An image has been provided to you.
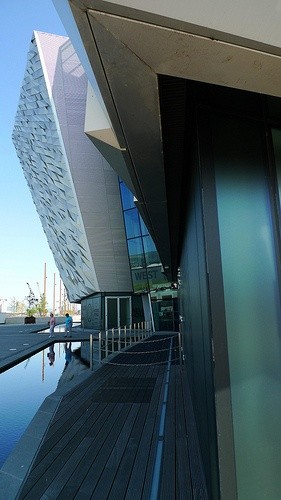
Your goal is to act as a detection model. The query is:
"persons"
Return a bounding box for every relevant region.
[47,343,55,366]
[48,313,56,338]
[63,313,73,338]
[65,341,72,366]
